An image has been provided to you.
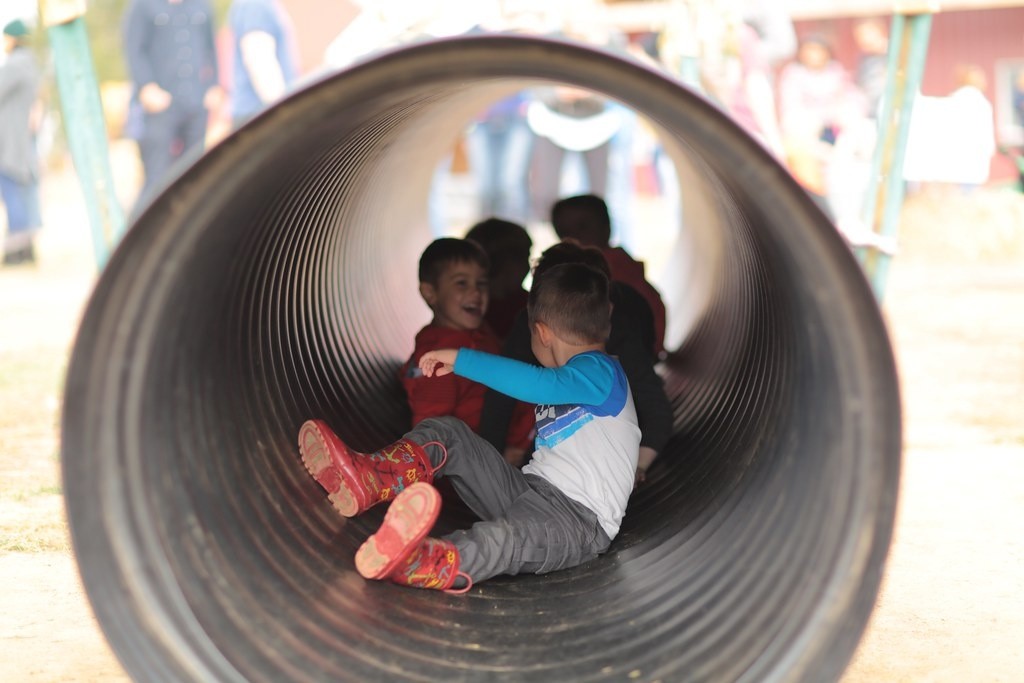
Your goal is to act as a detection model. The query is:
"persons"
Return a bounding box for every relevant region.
[648,0,1024,263]
[123,0,222,215]
[0,18,44,265]
[400,195,675,502]
[297,263,642,597]
[228,1,292,134]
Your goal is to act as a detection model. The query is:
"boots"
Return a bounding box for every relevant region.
[298,419,447,518]
[355,480,472,593]
